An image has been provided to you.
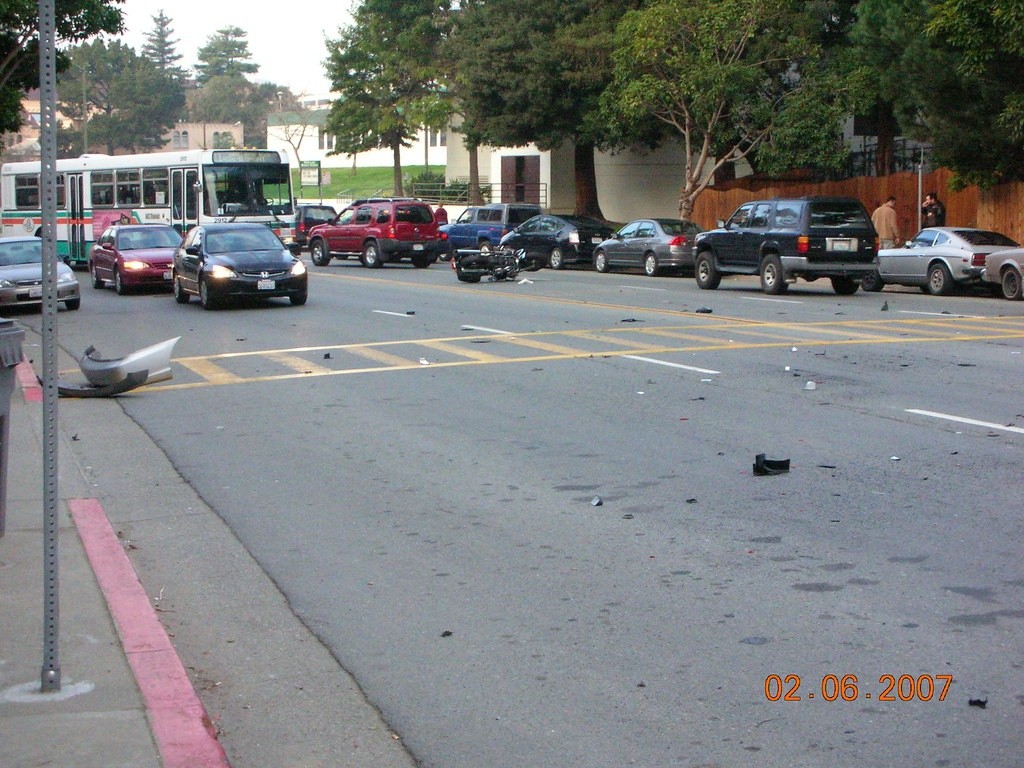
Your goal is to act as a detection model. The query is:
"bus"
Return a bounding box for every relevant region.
[1,148,299,264]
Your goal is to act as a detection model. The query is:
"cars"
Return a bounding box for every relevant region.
[591,218,707,277]
[88,223,184,296]
[499,213,616,271]
[862,226,1020,296]
[171,223,308,311]
[984,246,1023,301]
[0,235,81,317]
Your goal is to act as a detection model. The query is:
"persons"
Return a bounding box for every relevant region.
[434,203,448,225]
[871,196,900,249]
[921,192,945,230]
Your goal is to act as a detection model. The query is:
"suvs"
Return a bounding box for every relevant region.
[294,202,337,247]
[436,202,542,262]
[691,196,881,295]
[306,196,442,271]
